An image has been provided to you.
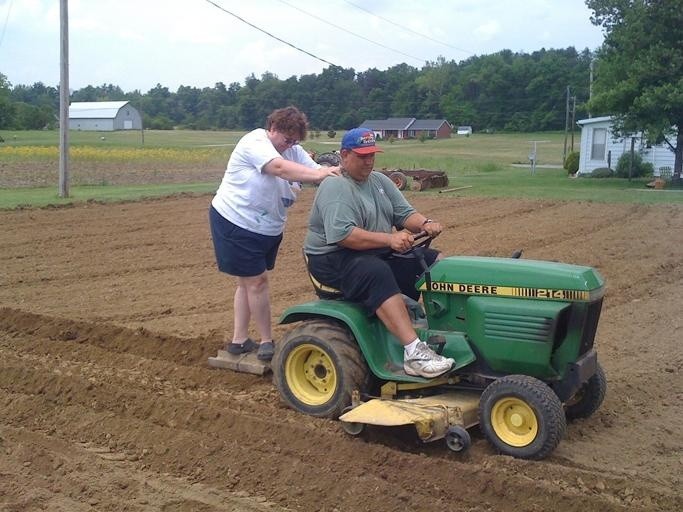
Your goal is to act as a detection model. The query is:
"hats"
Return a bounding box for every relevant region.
[341,127,384,155]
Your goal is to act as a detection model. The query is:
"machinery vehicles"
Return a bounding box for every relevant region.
[313,152,449,192]
[269,229,607,460]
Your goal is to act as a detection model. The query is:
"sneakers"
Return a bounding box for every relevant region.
[403,341,455,379]
[228,338,275,360]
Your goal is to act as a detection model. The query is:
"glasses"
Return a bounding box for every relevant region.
[279,135,299,145]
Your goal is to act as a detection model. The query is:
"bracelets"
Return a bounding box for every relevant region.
[423,218,432,225]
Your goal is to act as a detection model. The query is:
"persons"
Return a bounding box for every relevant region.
[207,105,342,358]
[303,128,457,378]
